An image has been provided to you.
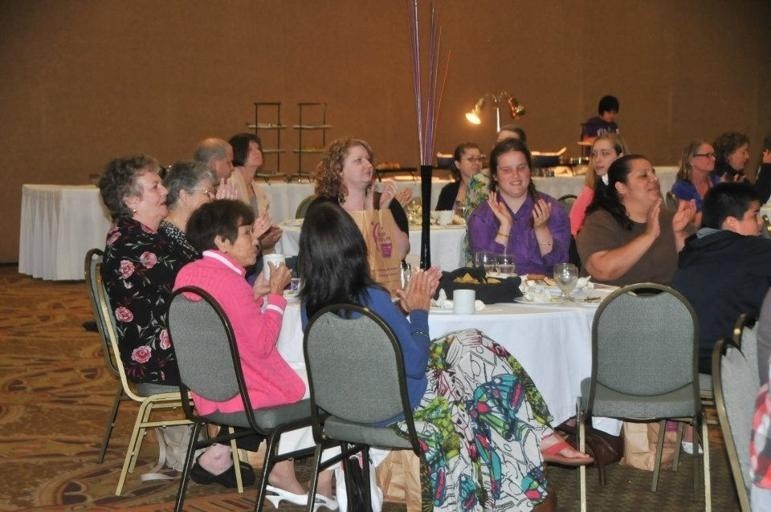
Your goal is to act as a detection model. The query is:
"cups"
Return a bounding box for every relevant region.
[476,252,516,277]
[454,288,476,314]
[263,254,287,283]
[438,211,453,226]
[291,279,301,292]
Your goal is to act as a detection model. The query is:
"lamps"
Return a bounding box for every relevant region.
[465,89,526,131]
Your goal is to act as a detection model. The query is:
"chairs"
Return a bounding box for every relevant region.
[82,248,137,463]
[571,282,712,512]
[731,313,765,389]
[94,262,244,496]
[706,337,760,512]
[302,300,430,512]
[164,286,326,512]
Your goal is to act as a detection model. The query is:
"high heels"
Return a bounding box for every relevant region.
[266,485,310,509]
[313,493,339,512]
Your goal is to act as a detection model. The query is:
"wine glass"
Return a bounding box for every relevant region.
[553,263,579,304]
[408,198,421,224]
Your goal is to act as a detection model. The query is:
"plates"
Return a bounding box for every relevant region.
[285,290,300,302]
[514,275,594,305]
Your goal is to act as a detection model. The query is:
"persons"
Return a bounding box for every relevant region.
[298,138,409,269]
[581,95,624,156]
[98,154,256,490]
[172,198,339,512]
[298,197,594,512]
[161,134,281,261]
[437,127,572,275]
[568,132,771,374]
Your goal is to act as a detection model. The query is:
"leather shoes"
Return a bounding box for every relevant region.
[192,452,255,488]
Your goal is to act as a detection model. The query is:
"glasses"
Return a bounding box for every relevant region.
[694,152,717,158]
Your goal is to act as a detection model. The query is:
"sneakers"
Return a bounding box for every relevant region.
[681,439,704,456]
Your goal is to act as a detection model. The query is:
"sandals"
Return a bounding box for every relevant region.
[541,432,595,465]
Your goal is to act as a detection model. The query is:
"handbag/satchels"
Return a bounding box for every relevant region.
[349,208,403,298]
[141,422,207,481]
[618,422,680,473]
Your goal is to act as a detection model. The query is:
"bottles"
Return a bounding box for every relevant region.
[403,264,413,284]
